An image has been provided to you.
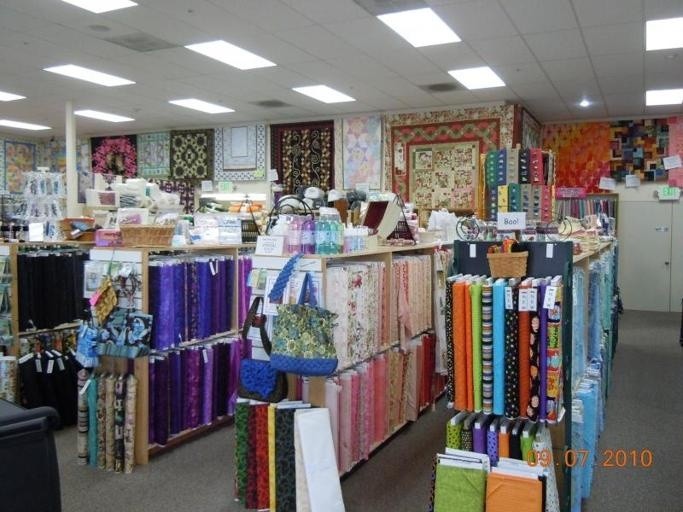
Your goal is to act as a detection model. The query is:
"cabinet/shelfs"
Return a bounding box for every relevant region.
[77,243,256,475]
[231,242,451,512]
[428,240,613,512]
[2,241,88,426]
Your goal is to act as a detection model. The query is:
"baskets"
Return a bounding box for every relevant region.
[122,224,176,248]
[487,251,529,278]
[57,218,95,241]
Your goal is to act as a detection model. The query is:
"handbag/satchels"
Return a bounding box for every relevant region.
[98,309,153,359]
[266,213,314,235]
[237,358,289,403]
[269,304,339,376]
[242,220,258,242]
[75,319,101,368]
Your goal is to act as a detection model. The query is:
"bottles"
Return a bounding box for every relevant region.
[286,207,344,256]
